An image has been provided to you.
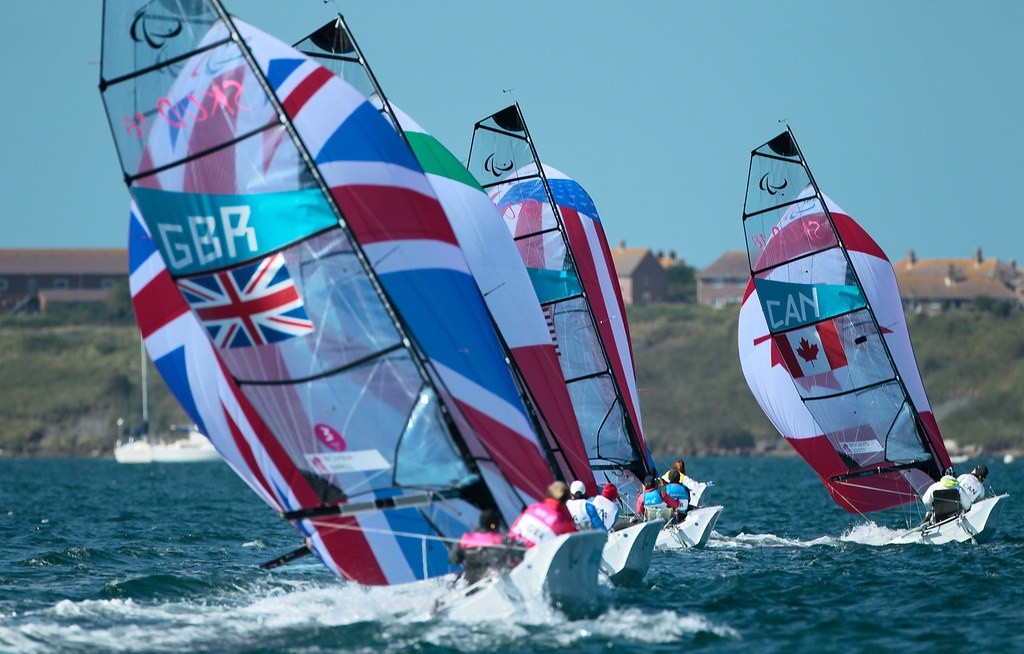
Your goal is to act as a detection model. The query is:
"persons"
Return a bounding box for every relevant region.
[508,481,579,548]
[959,464,989,504]
[923,466,972,526]
[586,482,620,531]
[660,468,691,523]
[565,480,609,534]
[448,510,534,596]
[636,476,682,522]
[662,460,687,486]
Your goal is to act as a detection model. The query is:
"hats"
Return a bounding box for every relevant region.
[602,483,617,500]
[668,469,680,483]
[644,476,654,489]
[549,481,568,500]
[481,510,499,527]
[570,480,586,495]
[976,464,988,479]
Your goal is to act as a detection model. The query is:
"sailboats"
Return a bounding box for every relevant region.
[99,0,609,619]
[737,120,1011,544]
[290,13,667,584]
[114,331,222,463]
[466,99,725,551]
